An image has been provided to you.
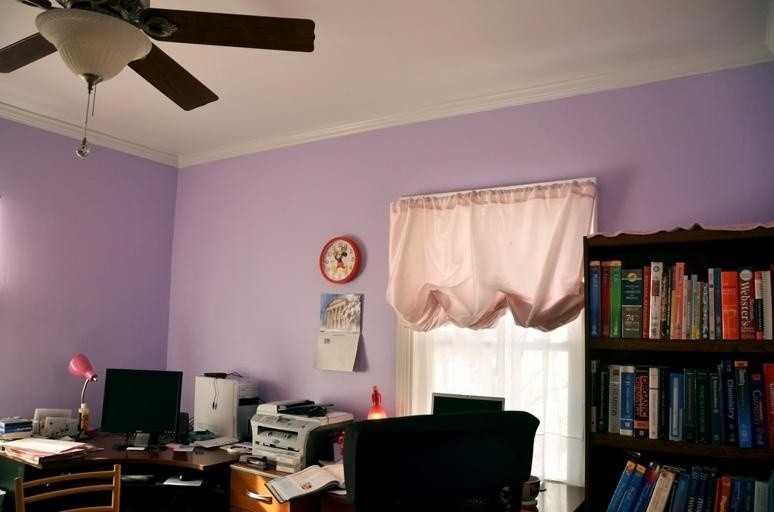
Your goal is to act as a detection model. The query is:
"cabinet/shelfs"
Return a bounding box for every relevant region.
[233,458,341,512]
[582,225,771,511]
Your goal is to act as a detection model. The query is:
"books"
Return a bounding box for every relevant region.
[1,437,105,465]
[262,464,346,504]
[586,260,773,342]
[589,357,774,449]
[606,460,772,511]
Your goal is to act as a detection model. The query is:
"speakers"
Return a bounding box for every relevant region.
[170,412,189,445]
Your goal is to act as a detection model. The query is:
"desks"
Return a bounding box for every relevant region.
[1,420,232,510]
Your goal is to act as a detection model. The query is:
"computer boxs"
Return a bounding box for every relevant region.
[193,377,258,440]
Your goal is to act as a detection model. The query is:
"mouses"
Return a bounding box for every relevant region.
[179,472,193,481]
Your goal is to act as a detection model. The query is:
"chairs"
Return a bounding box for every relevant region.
[10,464,123,510]
[343,408,541,512]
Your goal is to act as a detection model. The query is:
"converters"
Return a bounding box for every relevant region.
[205,372,228,378]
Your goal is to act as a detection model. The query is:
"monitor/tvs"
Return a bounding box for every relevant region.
[100,368,183,453]
[431,393,506,414]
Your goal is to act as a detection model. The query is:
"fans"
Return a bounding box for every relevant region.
[2,2,317,113]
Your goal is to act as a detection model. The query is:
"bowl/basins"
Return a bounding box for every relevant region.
[522,475,540,501]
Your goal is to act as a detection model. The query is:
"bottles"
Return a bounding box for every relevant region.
[78,403,89,431]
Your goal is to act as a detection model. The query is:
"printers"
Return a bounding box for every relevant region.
[250,397,354,474]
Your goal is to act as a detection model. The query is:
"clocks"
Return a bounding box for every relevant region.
[319,235,361,285]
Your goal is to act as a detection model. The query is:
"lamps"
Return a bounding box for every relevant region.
[31,6,155,158]
[66,351,97,438]
